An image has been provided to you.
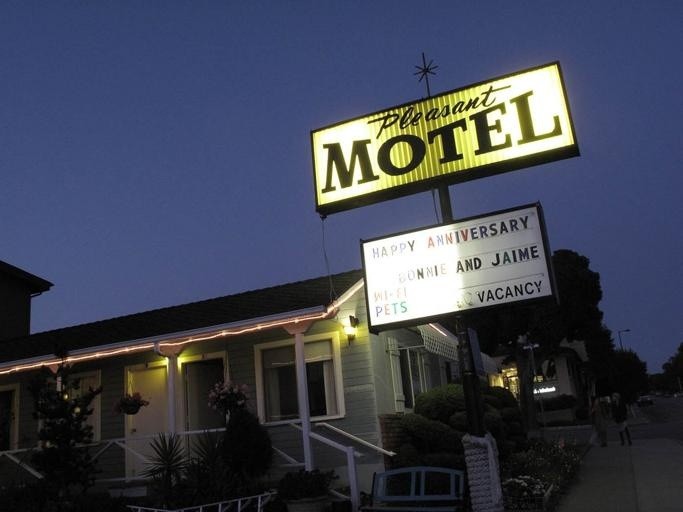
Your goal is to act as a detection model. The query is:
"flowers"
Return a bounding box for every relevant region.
[207,380,253,416]
[111,391,149,418]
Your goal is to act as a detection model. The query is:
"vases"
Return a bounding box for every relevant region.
[123,405,141,415]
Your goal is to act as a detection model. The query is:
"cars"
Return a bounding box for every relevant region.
[637,396,653,408]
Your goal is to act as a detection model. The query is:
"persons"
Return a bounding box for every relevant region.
[612,393,632,446]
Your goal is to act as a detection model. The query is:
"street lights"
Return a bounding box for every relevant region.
[522,344,546,437]
[618,328,630,353]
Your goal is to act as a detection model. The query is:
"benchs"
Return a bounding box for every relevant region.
[359,466,467,512]
